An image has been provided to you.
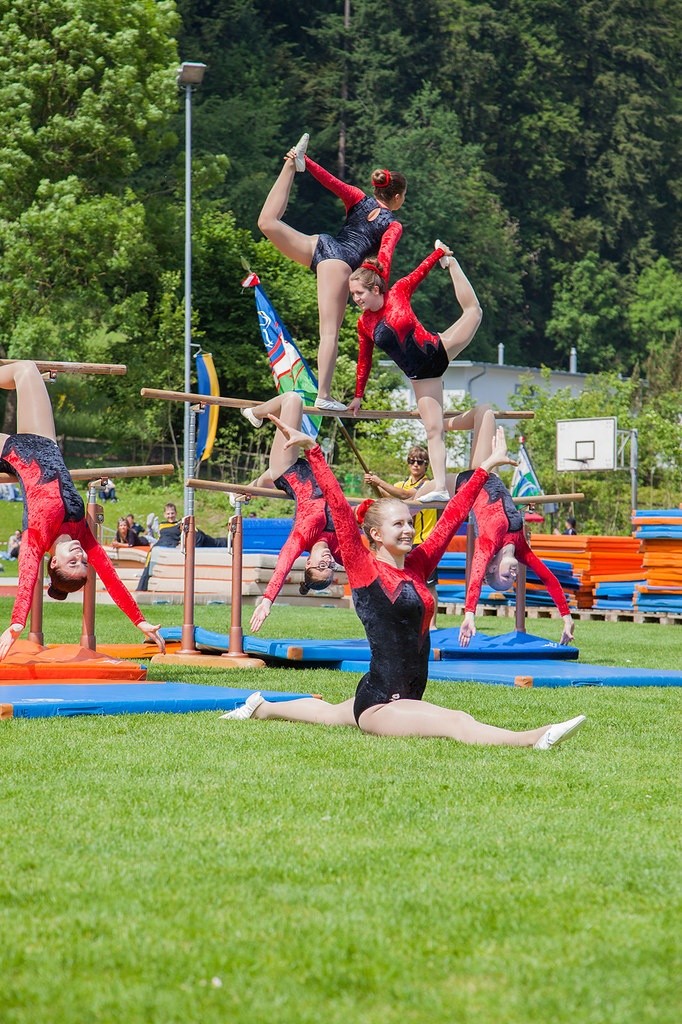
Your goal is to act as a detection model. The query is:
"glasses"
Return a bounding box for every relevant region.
[305,562,338,572]
[407,459,427,465]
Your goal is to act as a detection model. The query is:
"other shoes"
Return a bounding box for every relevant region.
[416,491,450,503]
[314,397,348,411]
[241,406,263,428]
[219,691,265,721]
[228,492,237,508]
[294,133,310,173]
[151,517,160,529]
[535,715,586,751]
[434,239,449,269]
[146,513,154,525]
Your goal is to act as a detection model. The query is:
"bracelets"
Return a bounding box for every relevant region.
[17,543,19,546]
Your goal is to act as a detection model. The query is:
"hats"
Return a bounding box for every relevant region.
[567,518,576,529]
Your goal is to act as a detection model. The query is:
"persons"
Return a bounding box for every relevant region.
[219,413,586,751]
[228,391,344,633]
[127,514,148,536]
[113,513,160,548]
[348,239,483,502]
[7,529,22,558]
[410,406,575,647]
[562,519,577,535]
[258,133,408,412]
[363,444,439,631]
[0,360,166,660]
[163,503,176,523]
[99,479,116,503]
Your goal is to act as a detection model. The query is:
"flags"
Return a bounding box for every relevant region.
[510,448,540,510]
[255,284,323,441]
